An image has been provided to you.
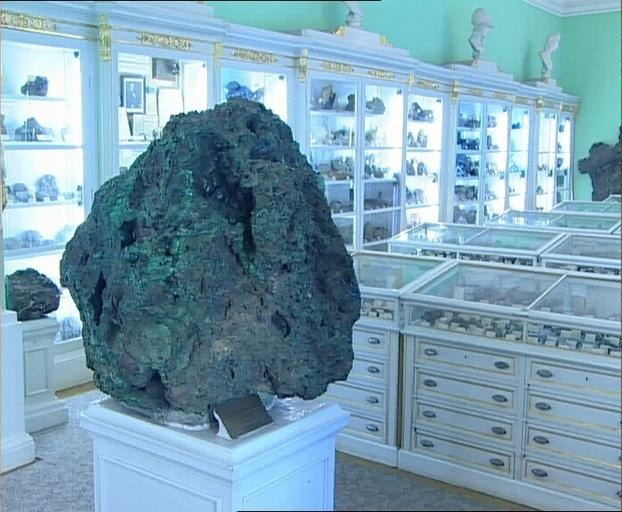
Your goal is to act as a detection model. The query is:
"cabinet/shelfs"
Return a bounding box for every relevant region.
[396,259,565,482]
[308,253,456,466]
[296,39,622,272]
[215,19,297,144]
[1,2,100,351]
[520,274,622,512]
[100,2,215,177]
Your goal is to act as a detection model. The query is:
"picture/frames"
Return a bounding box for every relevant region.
[152,57,178,83]
[119,74,147,116]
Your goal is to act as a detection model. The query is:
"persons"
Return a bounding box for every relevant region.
[540,33,560,76]
[470,7,494,60]
